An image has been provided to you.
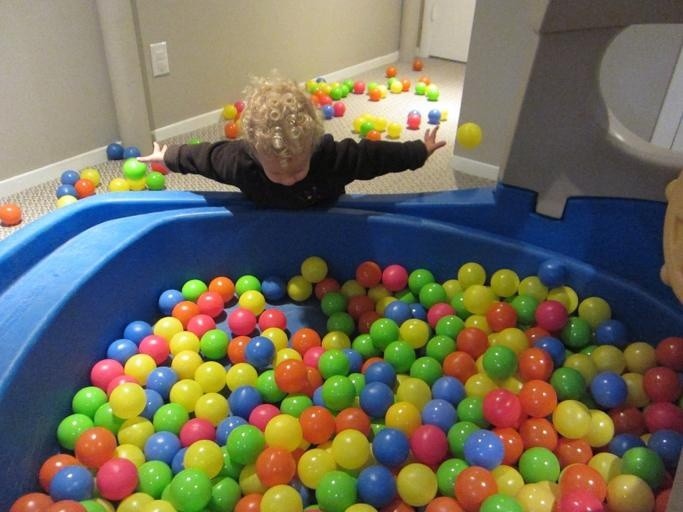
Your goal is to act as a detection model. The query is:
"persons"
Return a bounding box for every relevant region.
[137,76,449,208]
